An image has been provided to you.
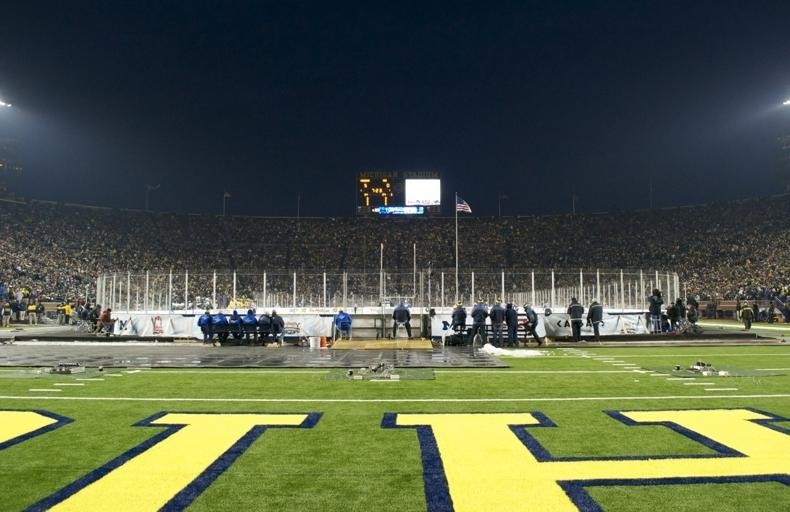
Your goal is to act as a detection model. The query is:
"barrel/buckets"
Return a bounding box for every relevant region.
[318,336,327,347]
[309,337,320,348]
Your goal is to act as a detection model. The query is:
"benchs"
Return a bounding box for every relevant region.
[448,322,530,348]
[211,323,284,348]
